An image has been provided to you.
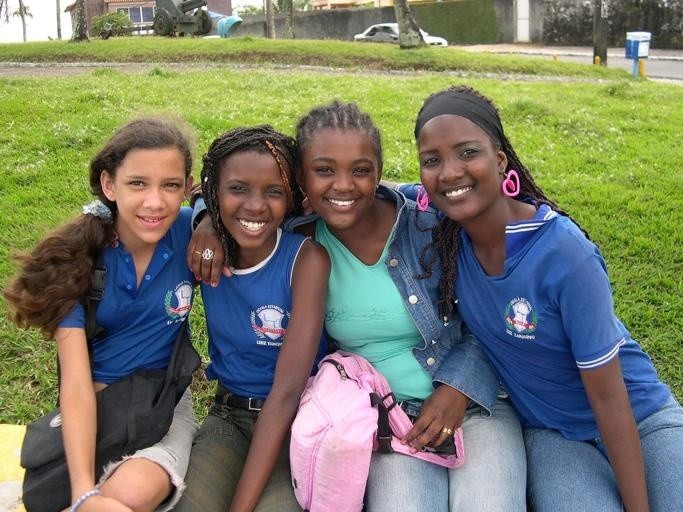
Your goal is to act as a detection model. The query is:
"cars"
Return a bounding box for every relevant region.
[354,21,448,48]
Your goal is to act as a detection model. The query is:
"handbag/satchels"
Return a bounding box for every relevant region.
[21,369,177,512]
[289,352,379,511]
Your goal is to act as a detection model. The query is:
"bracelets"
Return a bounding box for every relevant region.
[71,489,101,512]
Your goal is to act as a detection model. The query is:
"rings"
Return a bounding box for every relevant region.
[443,428,453,435]
[194,250,202,256]
[202,249,214,261]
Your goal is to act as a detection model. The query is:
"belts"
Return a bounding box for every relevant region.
[214,392,266,411]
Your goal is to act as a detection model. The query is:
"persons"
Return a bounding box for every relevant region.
[1,118,202,512]
[171,125,332,512]
[301,83,682,512]
[186,102,527,512]
